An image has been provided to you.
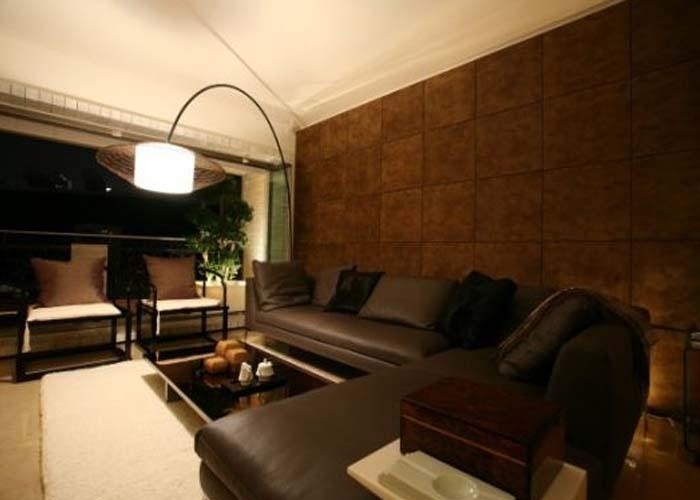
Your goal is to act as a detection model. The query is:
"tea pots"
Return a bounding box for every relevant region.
[239,359,274,386]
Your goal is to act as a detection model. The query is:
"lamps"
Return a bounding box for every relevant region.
[94,83,294,263]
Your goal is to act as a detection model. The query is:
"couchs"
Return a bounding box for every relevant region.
[194,270,651,500]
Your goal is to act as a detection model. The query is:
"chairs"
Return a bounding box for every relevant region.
[137,267,230,363]
[14,242,138,383]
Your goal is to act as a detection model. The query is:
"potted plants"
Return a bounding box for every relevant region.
[184,177,254,316]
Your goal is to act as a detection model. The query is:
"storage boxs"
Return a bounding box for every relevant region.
[398,376,570,500]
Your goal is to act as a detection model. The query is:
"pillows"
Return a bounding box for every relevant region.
[435,269,518,350]
[322,267,389,314]
[141,253,202,301]
[496,289,592,382]
[251,260,313,311]
[28,255,115,308]
[355,272,456,330]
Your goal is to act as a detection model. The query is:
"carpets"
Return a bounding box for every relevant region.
[38,357,239,500]
